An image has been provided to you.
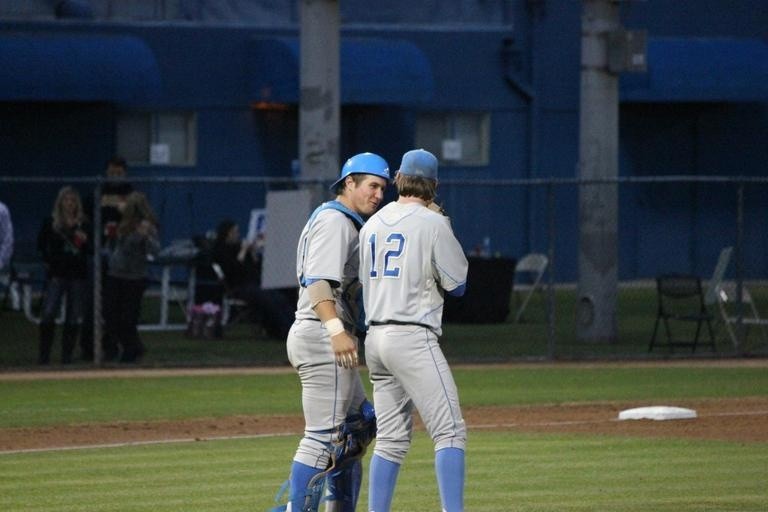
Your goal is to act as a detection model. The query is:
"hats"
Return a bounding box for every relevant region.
[398,149,437,179]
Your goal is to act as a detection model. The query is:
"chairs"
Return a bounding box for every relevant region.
[509,249,552,325]
[714,278,768,350]
[0,235,249,336]
[647,271,718,357]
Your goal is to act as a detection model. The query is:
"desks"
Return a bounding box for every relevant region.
[439,253,516,325]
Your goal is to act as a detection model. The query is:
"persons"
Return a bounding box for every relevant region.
[359,148,470,512]
[37,156,160,364]
[285,152,390,512]
[0,201,15,272]
[210,221,295,337]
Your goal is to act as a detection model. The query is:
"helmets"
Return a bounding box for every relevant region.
[329,153,389,194]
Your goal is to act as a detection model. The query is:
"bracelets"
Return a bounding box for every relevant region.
[323,318,345,338]
